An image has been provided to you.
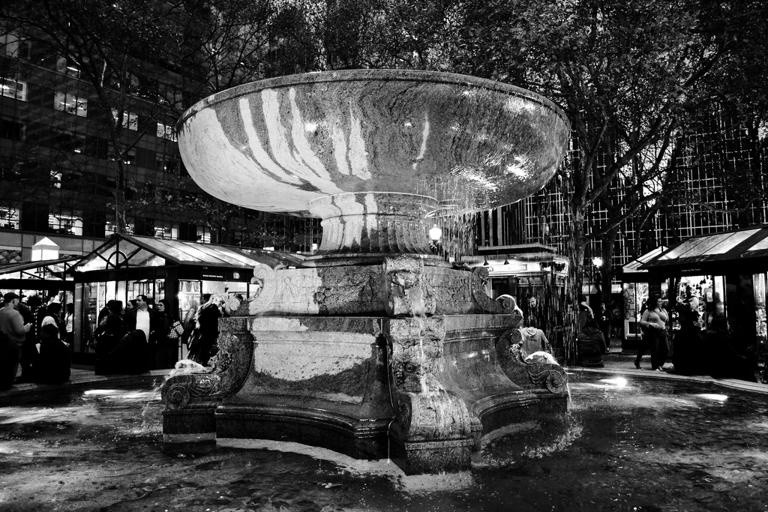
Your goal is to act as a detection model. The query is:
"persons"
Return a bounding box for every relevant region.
[524,288,730,378]
[0,290,245,396]
